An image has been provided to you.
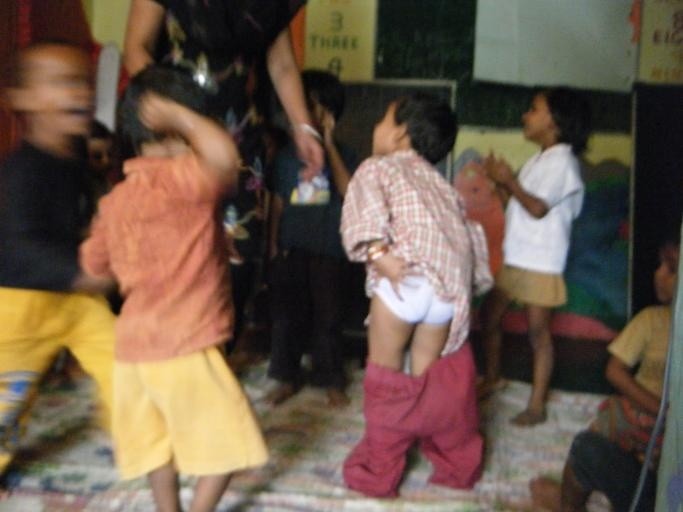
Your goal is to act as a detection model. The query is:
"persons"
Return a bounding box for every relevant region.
[2,40,119,483]
[123,2,328,358]
[340,91,493,496]
[78,59,268,511]
[266,68,356,408]
[562,241,678,512]
[46,120,112,389]
[0,2,589,512]
[477,85,590,423]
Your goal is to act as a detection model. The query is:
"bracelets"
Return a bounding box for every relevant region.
[301,123,322,142]
[369,247,389,261]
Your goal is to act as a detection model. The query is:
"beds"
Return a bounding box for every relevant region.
[0,350,612,512]
